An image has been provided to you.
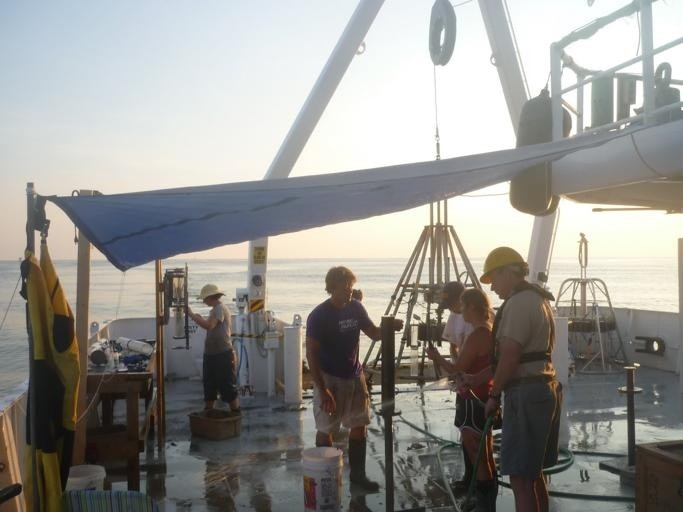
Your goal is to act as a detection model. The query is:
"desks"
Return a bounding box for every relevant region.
[88,338,160,491]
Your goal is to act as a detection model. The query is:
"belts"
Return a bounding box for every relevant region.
[503,375,553,385]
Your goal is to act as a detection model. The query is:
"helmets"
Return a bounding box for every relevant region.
[197,285,225,301]
[479,246,527,283]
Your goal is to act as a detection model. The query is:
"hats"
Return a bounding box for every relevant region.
[439,281,463,309]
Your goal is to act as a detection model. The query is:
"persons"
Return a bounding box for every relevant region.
[440,282,475,492]
[426,288,498,511]
[307,266,403,492]
[480,247,563,511]
[183,284,241,414]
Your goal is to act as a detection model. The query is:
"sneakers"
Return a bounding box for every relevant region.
[451,481,473,489]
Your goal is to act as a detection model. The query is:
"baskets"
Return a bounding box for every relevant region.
[187,408,242,440]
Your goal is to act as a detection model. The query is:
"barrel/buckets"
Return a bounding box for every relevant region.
[301,447,344,512]
[64,465,107,491]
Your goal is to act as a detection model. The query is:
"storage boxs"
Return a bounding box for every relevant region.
[189,413,242,438]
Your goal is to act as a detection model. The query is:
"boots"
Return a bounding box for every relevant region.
[477,480,495,512]
[349,439,377,490]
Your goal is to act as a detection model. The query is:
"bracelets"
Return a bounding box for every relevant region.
[487,389,503,400]
